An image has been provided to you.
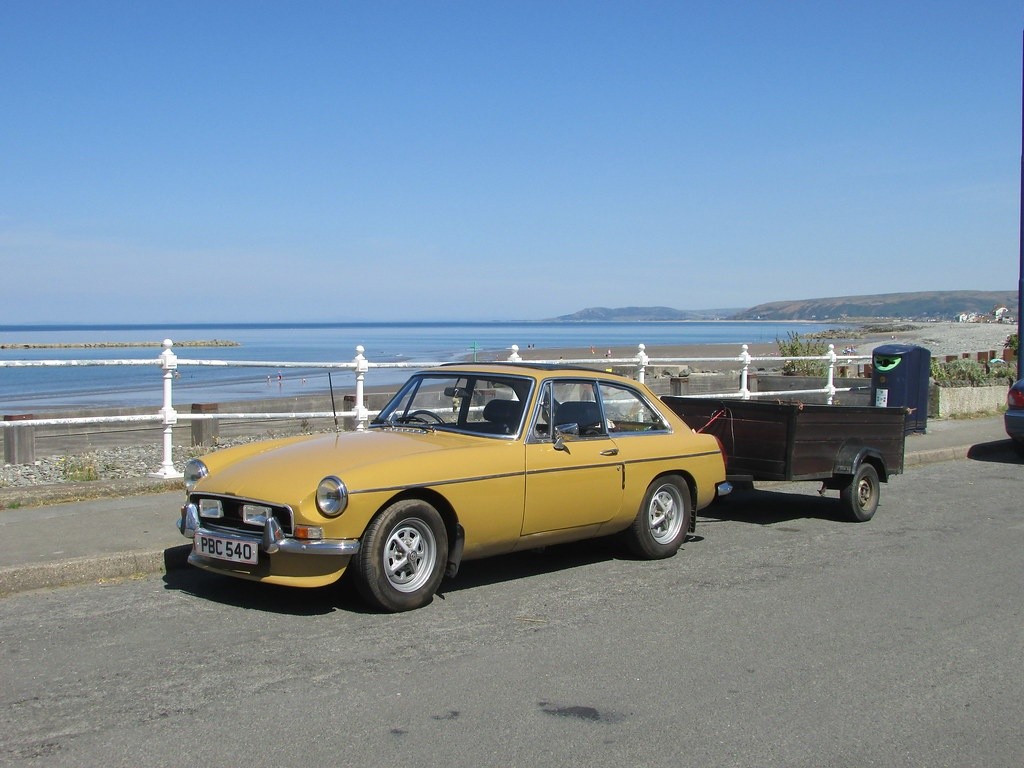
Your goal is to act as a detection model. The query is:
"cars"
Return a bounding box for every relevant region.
[1003,378,1024,447]
[176,358,730,616]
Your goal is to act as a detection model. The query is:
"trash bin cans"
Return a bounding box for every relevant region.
[869,343,931,438]
